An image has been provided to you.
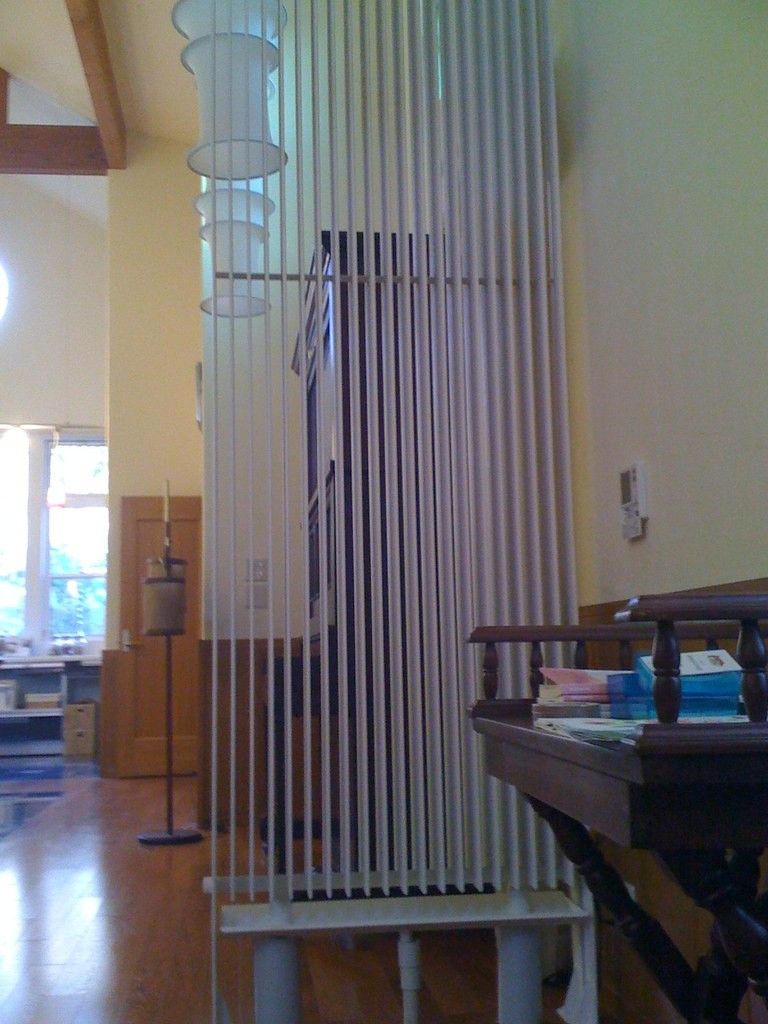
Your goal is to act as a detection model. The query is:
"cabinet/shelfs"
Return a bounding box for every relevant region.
[1,656,68,756]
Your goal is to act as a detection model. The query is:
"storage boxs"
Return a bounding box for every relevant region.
[63,700,100,763]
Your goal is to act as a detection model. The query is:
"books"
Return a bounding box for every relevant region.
[531,646,754,746]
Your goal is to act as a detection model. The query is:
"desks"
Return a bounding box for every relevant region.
[463,594,768,1024]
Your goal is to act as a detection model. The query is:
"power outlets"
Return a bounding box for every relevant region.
[245,557,269,581]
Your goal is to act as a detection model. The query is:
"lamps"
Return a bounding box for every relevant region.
[172,1,290,319]
